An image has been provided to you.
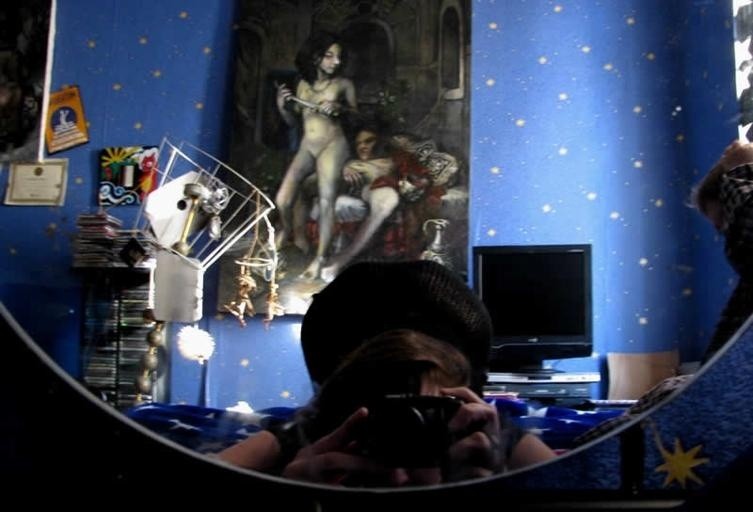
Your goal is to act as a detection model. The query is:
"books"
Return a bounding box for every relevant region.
[73,212,158,411]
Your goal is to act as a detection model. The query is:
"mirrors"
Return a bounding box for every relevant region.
[0,0,752,492]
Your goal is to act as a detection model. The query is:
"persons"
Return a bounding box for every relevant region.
[691,137,751,367]
[208,257,556,490]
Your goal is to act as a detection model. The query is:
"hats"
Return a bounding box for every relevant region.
[300,259,491,391]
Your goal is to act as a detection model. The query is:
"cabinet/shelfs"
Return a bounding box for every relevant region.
[70,264,170,411]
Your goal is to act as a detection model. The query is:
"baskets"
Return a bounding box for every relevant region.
[135,135,275,269]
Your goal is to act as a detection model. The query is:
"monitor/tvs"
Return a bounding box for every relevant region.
[473,244,593,375]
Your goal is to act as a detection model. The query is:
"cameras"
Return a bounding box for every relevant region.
[371,395,467,468]
